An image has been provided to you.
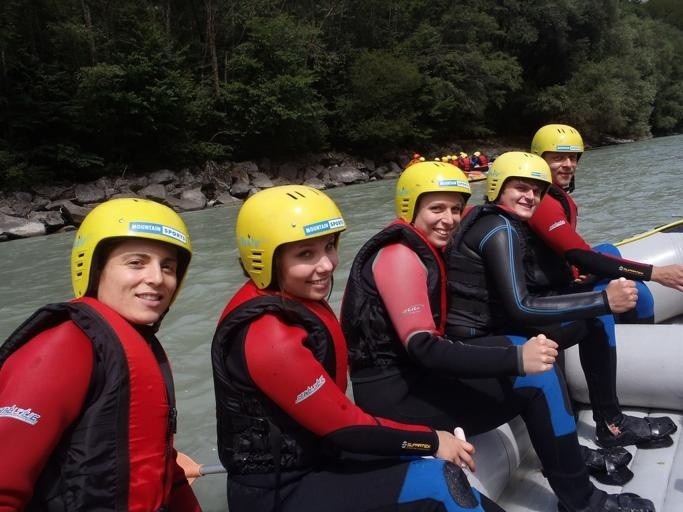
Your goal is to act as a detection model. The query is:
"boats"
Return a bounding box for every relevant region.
[421,221,681,512]
[464,170,487,182]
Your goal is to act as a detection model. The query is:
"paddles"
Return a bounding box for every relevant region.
[465,170,481,175]
[177,427,472,485]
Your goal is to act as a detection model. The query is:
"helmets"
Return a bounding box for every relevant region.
[486,151,553,203]
[237,185,347,290]
[414,151,481,162]
[531,124,584,160]
[71,197,191,307]
[396,161,471,225]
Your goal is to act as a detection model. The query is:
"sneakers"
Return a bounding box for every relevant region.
[595,415,677,445]
[578,445,633,485]
[590,492,655,511]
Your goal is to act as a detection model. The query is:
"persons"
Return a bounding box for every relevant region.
[0,197,203,511]
[440,152,678,487]
[209,184,503,510]
[409,152,492,172]
[527,121,682,324]
[338,160,657,511]
